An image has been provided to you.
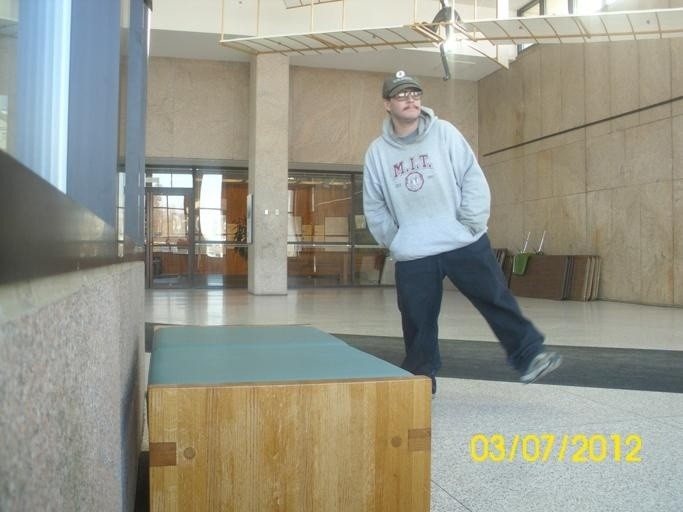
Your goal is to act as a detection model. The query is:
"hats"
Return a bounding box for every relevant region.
[382,75,423,99]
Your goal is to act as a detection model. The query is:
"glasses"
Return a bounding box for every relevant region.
[393,87,422,102]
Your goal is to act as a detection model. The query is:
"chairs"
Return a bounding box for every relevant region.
[511,228,547,276]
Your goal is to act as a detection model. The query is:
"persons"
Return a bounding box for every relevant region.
[360,67,565,398]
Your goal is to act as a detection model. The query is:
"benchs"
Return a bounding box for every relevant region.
[144,322,433,511]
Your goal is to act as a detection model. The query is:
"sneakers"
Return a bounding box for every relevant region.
[519,350,562,385]
